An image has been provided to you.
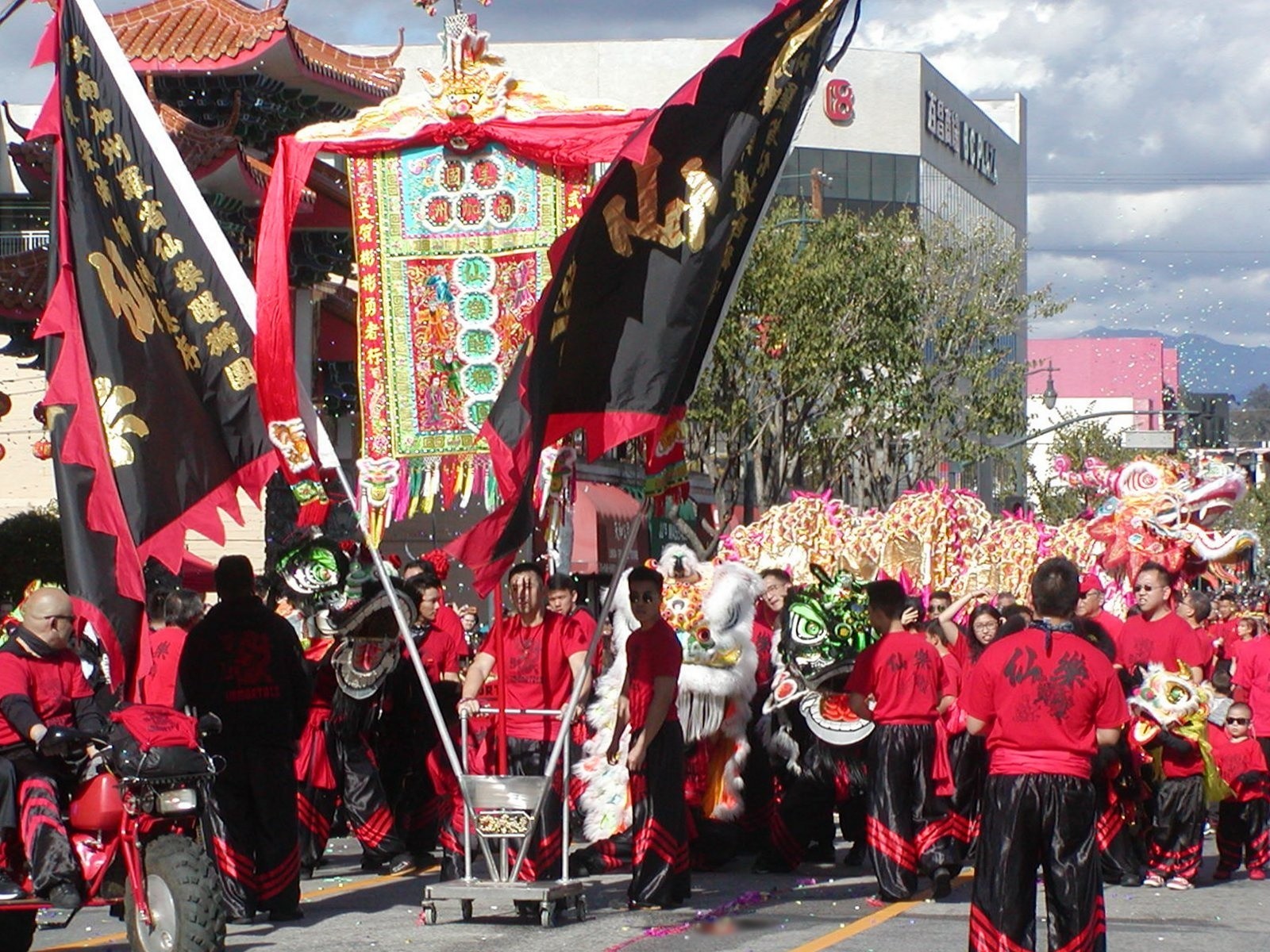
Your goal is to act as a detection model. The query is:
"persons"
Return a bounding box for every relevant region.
[178,554,313,925]
[0,565,1270,952]
[956,557,1132,952]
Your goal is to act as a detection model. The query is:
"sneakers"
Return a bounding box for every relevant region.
[49,883,80,908]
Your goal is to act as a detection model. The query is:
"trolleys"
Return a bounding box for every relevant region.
[420,708,588,928]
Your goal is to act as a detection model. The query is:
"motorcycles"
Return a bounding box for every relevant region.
[0,698,229,952]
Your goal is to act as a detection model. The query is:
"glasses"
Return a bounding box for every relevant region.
[1134,585,1167,592]
[1080,592,1099,599]
[45,615,78,624]
[629,594,661,603]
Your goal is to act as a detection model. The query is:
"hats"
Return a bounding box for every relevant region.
[1078,573,1105,594]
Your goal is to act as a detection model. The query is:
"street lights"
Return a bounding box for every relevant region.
[976,362,1062,498]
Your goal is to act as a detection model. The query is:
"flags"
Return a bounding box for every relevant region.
[28,0,338,706]
[443,0,860,599]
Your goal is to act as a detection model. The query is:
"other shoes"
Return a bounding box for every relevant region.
[1121,871,1142,886]
[362,857,380,870]
[415,852,443,873]
[389,854,417,876]
[1249,868,1265,880]
[1143,874,1165,887]
[660,897,682,908]
[844,842,866,866]
[270,908,304,921]
[934,868,952,900]
[750,859,769,874]
[1166,876,1195,891]
[1213,870,1230,879]
[229,916,253,925]
[0,873,27,900]
